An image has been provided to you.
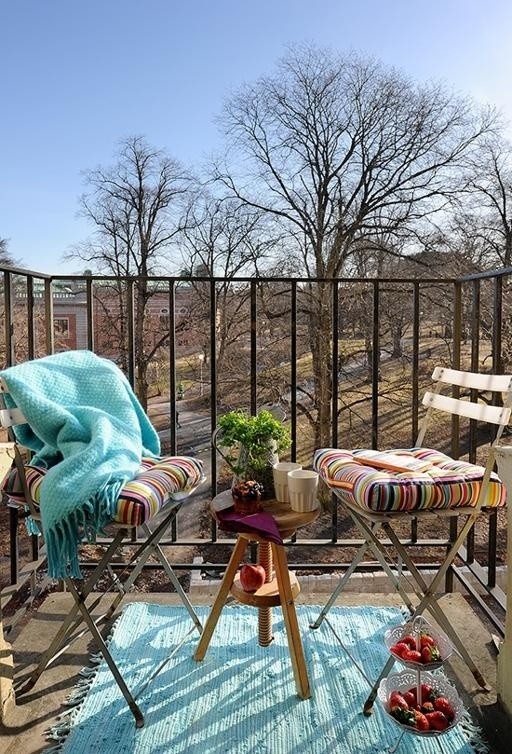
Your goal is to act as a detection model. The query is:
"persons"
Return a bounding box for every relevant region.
[176,410,182,428]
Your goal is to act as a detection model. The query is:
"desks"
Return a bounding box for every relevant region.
[192,488,321,699]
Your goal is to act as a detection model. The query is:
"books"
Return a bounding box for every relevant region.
[353,446,433,475]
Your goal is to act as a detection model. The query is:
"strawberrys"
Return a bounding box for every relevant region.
[389,683,455,734]
[389,633,436,664]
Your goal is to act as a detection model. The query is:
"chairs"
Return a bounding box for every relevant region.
[308,366,512,717]
[1,370,204,727]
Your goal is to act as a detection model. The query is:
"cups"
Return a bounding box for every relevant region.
[287,469,319,513]
[272,462,302,503]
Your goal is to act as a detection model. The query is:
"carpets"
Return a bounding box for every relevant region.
[41,603,492,753]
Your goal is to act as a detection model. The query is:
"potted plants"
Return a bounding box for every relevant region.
[217,408,294,497]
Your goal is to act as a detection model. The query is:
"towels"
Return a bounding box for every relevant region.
[215,501,282,545]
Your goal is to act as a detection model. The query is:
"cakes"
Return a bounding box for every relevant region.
[231,478,264,516]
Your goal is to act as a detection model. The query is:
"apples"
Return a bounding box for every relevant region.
[240,564,265,593]
